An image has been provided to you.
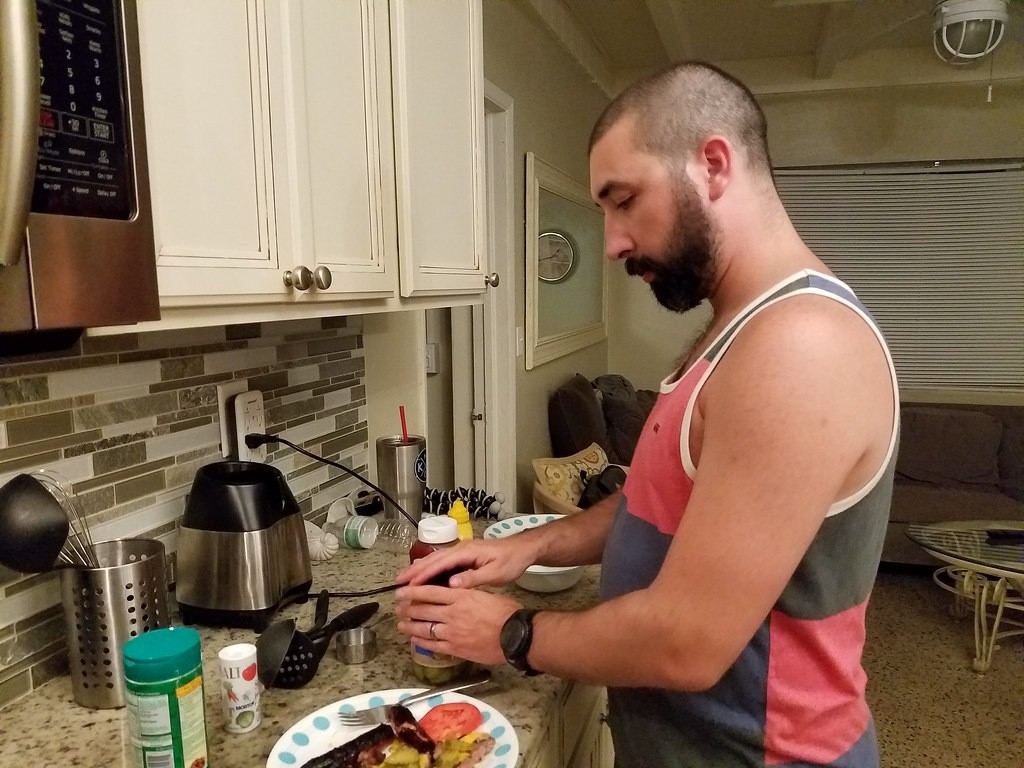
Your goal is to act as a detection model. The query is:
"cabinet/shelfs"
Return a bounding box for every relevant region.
[86,0,397,337]
[381,0,500,314]
[521,680,615,768]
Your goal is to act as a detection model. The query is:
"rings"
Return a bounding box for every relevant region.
[430,621,440,642]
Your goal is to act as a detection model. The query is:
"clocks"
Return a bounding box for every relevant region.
[537,227,580,285]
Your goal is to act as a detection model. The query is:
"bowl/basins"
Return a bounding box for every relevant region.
[480,511,590,594]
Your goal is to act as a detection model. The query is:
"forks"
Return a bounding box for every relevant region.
[339,672,497,728]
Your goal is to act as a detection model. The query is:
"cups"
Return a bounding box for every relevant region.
[375,432,427,525]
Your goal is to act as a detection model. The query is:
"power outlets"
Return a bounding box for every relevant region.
[216,382,268,463]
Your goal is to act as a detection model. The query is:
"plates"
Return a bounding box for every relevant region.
[265,687,524,768]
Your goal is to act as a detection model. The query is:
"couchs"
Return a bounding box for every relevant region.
[880,402,1024,568]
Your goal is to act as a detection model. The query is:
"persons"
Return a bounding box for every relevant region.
[392,61,900,768]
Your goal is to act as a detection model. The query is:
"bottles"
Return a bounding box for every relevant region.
[217,643,261,736]
[323,514,416,555]
[407,495,473,682]
[121,626,211,768]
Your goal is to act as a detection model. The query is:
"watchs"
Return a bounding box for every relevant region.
[500,608,544,677]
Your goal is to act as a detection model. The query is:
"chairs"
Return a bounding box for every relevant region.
[532,464,631,516]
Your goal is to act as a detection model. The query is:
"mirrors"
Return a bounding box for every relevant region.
[523,150,610,372]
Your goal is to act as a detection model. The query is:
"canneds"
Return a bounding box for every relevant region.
[409,598,472,684]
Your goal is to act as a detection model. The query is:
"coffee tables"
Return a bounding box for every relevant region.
[902,514,1024,675]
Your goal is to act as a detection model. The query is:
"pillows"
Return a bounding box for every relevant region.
[894,407,1003,486]
[548,372,608,457]
[602,392,648,463]
[531,442,609,506]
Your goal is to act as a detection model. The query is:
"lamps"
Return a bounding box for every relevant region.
[928,0,1011,68]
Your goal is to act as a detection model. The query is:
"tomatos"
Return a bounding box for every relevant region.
[418,702,483,742]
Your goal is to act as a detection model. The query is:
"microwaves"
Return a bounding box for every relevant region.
[1,0,162,339]
